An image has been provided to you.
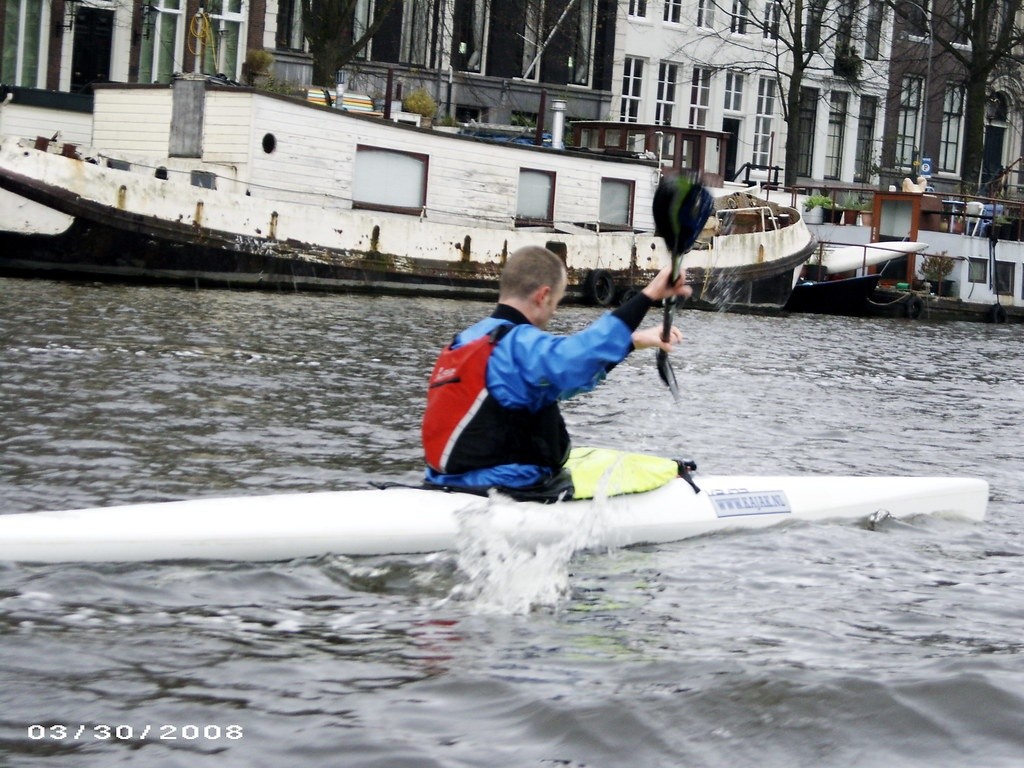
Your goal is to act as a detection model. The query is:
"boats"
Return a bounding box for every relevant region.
[0,62,817,318]
[0,471,989,566]
[785,240,931,317]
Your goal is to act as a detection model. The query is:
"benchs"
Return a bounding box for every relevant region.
[390,100,421,127]
[301,88,384,116]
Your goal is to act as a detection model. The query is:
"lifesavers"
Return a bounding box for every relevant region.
[617,288,639,306]
[992,303,1009,324]
[588,269,615,306]
[906,296,926,320]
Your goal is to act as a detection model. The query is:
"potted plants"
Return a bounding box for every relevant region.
[403,84,438,128]
[802,195,874,226]
[984,213,1019,241]
[432,117,460,133]
[918,250,956,297]
[800,243,831,281]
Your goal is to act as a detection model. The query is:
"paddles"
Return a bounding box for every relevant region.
[652,176,716,404]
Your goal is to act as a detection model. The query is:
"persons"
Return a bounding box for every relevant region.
[423,245,692,502]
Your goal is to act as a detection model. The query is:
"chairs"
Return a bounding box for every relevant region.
[958,201,984,236]
